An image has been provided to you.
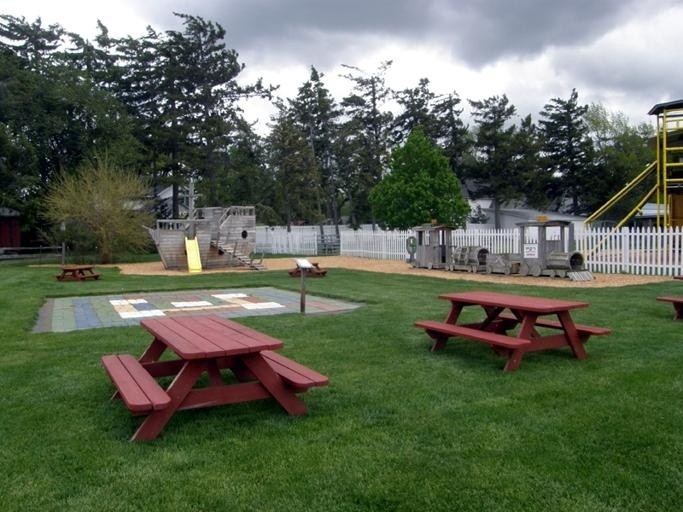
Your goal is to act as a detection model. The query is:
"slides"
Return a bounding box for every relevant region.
[185,236,203,273]
[579,160,658,265]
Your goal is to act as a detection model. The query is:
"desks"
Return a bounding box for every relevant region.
[292,263,323,277]
[59,265,97,280]
[437,289,588,372]
[131,311,304,443]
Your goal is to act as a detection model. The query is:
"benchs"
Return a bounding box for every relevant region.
[52,272,100,281]
[288,270,328,279]
[100,350,330,444]
[412,312,612,373]
